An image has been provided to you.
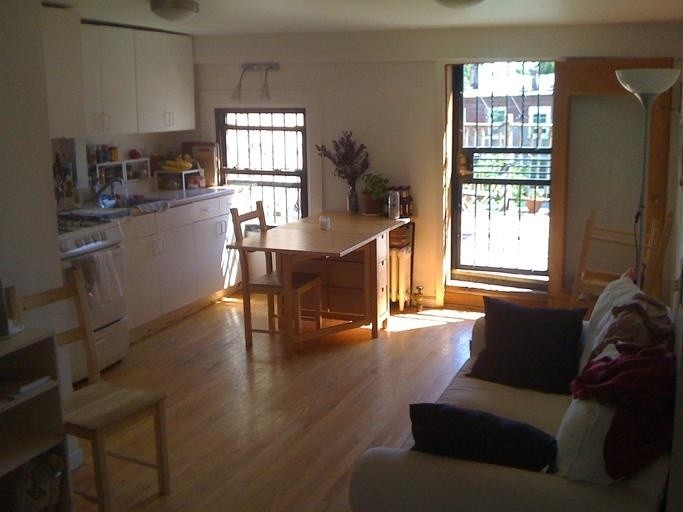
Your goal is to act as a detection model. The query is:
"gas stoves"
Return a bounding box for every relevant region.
[58,213,113,235]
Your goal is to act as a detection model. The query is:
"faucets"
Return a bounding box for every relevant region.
[91,176,122,202]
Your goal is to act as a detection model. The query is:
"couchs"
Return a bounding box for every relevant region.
[348,277,683,512]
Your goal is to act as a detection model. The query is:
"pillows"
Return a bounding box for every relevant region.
[409,403,557,473]
[465,296,588,396]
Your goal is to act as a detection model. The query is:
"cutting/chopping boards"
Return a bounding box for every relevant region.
[180,140,221,190]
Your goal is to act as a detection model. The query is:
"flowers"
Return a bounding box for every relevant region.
[314,129,369,186]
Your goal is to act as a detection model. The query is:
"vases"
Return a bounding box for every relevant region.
[346,186,358,214]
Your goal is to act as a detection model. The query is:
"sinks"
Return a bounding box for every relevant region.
[95,198,161,209]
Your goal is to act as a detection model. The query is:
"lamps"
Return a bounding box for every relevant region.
[259,66,272,100]
[615,69,681,291]
[230,66,250,100]
[150,0,199,23]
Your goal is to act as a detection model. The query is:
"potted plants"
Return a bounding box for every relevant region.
[527,191,541,213]
[357,172,390,217]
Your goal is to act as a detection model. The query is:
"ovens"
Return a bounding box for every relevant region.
[56,224,129,384]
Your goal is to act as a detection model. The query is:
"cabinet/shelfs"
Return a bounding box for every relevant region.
[81,24,138,139]
[194,213,245,294]
[135,30,195,134]
[0,328,73,512]
[59,188,252,242]
[42,3,85,138]
[157,223,199,315]
[125,234,162,330]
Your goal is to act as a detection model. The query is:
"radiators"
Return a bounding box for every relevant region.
[389,244,436,312]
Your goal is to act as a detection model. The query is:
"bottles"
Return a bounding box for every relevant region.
[400,188,408,217]
[408,185,413,217]
[88,167,97,185]
[389,192,400,219]
[86,143,119,163]
[127,163,149,180]
[384,192,388,216]
[100,166,122,183]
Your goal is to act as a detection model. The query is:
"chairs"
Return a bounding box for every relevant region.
[4,268,171,512]
[230,201,328,353]
